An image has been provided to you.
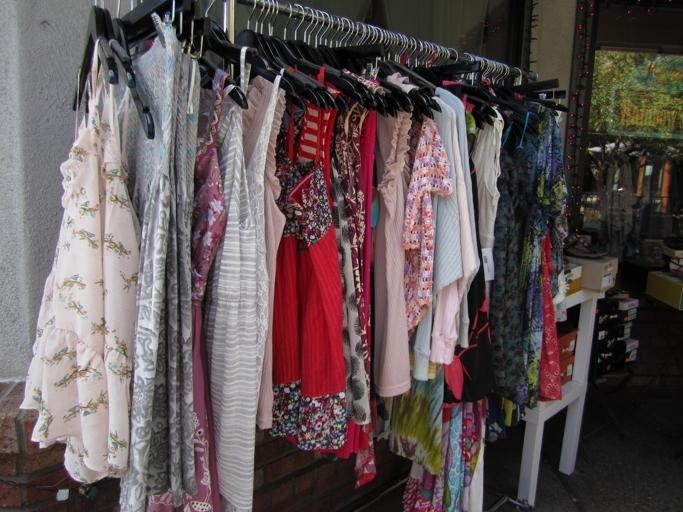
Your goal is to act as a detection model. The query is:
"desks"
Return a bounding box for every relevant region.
[512,287,606,509]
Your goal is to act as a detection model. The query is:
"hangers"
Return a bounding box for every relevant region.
[72,1,570,141]
